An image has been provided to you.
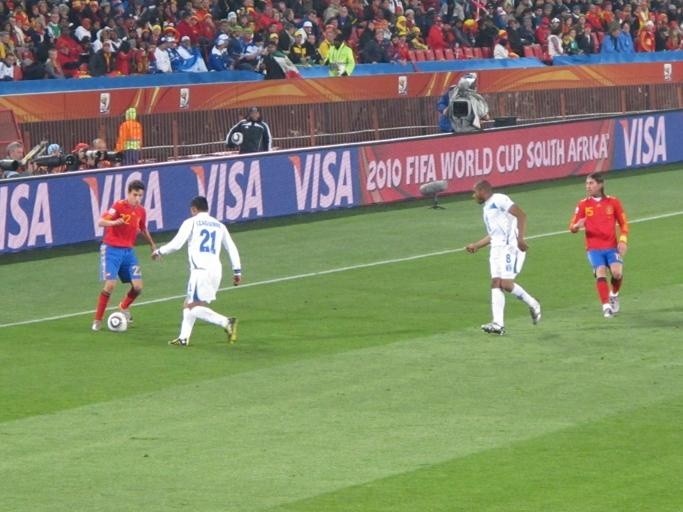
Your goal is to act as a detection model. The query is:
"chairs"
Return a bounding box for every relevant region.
[405,44,552,65]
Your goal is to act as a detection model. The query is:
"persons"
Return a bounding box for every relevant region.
[90,180,154,331]
[2,138,112,178]
[2,2,682,81]
[462,179,542,335]
[436,82,459,133]
[150,196,242,349]
[224,105,273,155]
[570,173,628,319]
[115,107,144,165]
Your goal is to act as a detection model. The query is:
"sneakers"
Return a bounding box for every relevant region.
[225,317,237,344]
[169,337,189,346]
[604,295,619,318]
[118,302,135,323]
[92,319,101,331]
[482,322,505,334]
[530,300,541,324]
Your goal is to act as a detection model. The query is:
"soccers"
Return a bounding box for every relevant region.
[107,312,127,333]
[231,132,243,145]
